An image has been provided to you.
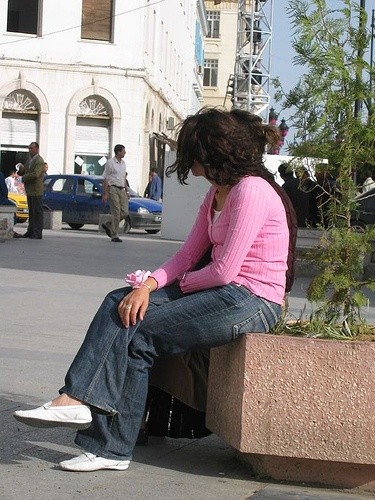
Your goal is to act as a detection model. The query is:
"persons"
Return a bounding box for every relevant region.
[5,169,17,194]
[144,168,162,203]
[132,108,297,447]
[0,171,23,238]
[12,103,289,471]
[278,162,375,227]
[19,142,44,238]
[44,163,48,175]
[102,144,131,242]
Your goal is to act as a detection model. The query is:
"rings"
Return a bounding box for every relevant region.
[125,304,131,309]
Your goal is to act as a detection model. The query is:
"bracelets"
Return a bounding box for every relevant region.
[142,283,151,292]
[126,191,130,194]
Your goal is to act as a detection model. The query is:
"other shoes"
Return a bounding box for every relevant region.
[102,223,112,237]
[111,237,122,242]
[13,232,23,238]
[23,232,42,239]
[135,428,149,446]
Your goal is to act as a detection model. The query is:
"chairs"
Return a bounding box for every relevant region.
[78,185,85,193]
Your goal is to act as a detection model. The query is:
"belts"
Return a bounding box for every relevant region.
[111,185,125,190]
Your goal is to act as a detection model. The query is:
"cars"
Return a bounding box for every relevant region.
[43,174,162,234]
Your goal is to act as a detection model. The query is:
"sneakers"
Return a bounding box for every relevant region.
[59,450,130,471]
[13,399,92,430]
